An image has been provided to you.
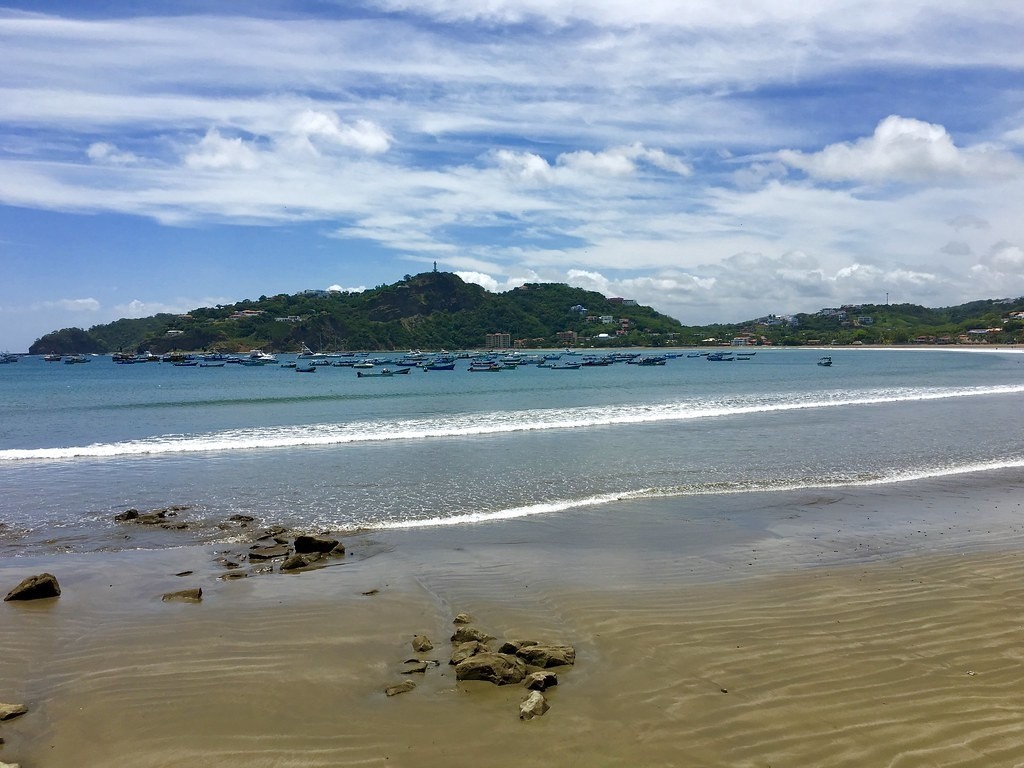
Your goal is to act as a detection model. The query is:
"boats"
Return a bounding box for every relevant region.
[543,353,562,360]
[356,368,393,378]
[551,361,581,369]
[171,358,191,364]
[280,360,296,368]
[817,356,832,366]
[239,359,264,366]
[44,354,62,361]
[350,359,374,368]
[297,342,327,359]
[416,361,437,368]
[536,363,554,368]
[373,357,398,366]
[174,361,199,366]
[200,362,225,367]
[332,359,360,367]
[248,350,279,364]
[714,351,733,355]
[687,353,710,358]
[625,353,684,366]
[226,357,247,363]
[163,344,584,366]
[64,356,91,364]
[308,360,333,366]
[295,366,316,372]
[396,360,423,366]
[393,367,410,374]
[112,351,160,364]
[467,363,501,372]
[581,352,641,366]
[706,354,734,361]
[737,356,750,360]
[0,353,20,363]
[422,362,456,372]
[737,351,756,355]
